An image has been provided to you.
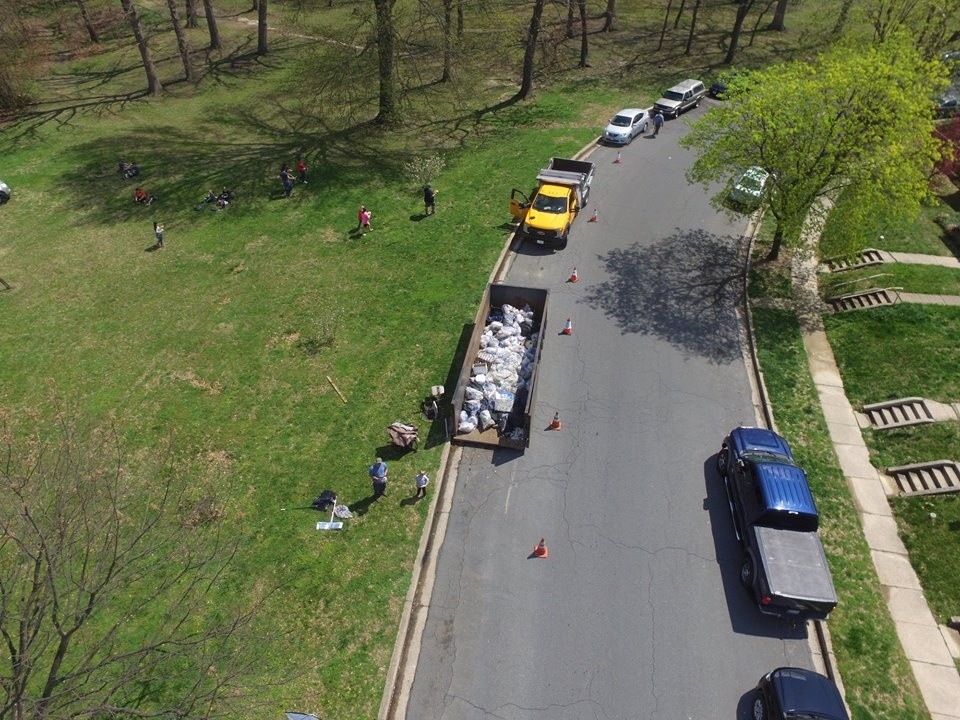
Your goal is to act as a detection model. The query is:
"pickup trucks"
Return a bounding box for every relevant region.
[509,155,597,250]
[716,426,839,629]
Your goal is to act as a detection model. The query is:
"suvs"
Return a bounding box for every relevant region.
[652,78,706,119]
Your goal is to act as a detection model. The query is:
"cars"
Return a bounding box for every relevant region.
[602,108,650,145]
[921,46,960,114]
[709,81,742,101]
[729,165,769,210]
[752,666,850,720]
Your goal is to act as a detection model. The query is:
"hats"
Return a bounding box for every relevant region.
[376,458,382,464]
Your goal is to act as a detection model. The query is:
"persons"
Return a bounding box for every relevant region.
[153,222,165,246]
[416,471,429,498]
[297,159,308,184]
[423,184,438,216]
[279,161,297,198]
[358,205,374,236]
[120,160,139,178]
[653,110,664,137]
[135,189,147,203]
[369,458,388,503]
[207,186,232,206]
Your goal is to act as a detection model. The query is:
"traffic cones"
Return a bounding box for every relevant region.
[568,266,579,283]
[549,410,562,431]
[561,317,572,336]
[534,538,548,558]
[614,151,622,164]
[589,209,599,222]
